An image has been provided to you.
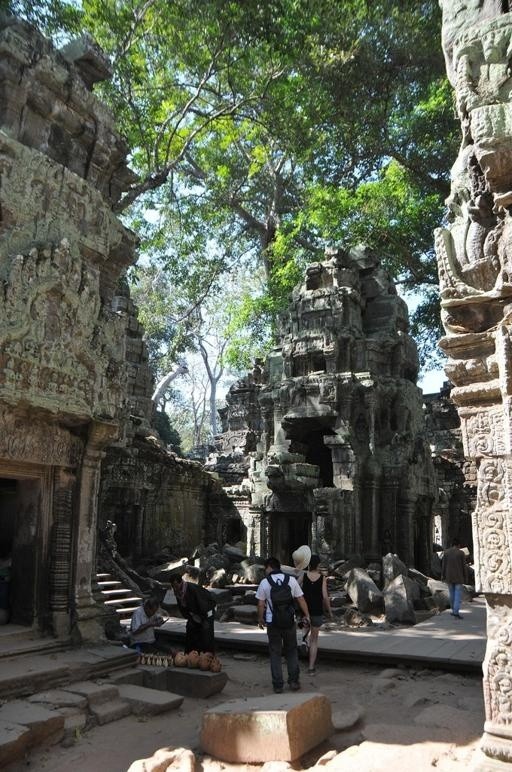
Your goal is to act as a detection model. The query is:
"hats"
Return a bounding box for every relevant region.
[292,544,312,570]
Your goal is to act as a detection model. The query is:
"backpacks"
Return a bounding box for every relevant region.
[265,573,295,629]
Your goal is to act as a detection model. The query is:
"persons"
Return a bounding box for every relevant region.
[440,538,471,621]
[129,596,177,660]
[295,554,336,675]
[254,557,311,694]
[174,582,220,659]
